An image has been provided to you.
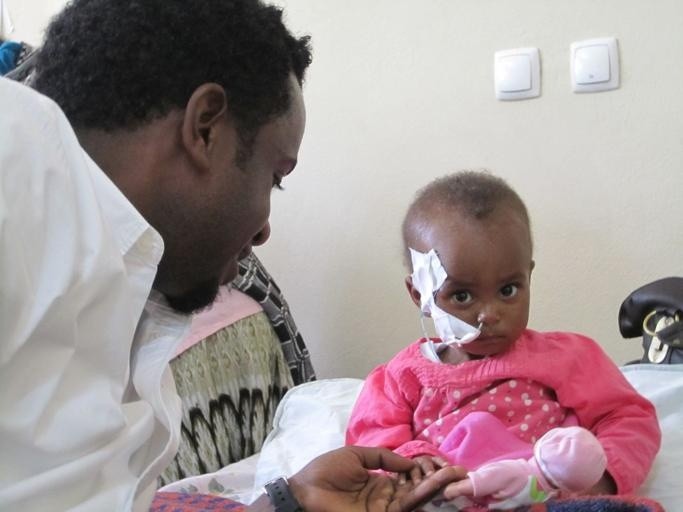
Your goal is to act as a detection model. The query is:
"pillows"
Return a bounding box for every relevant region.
[254,360,683,511]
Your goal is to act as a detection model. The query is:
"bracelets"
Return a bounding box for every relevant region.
[262,473,303,512]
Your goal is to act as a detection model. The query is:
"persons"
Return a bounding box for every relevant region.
[437,425,607,509]
[1,0,468,512]
[344,172,661,512]
